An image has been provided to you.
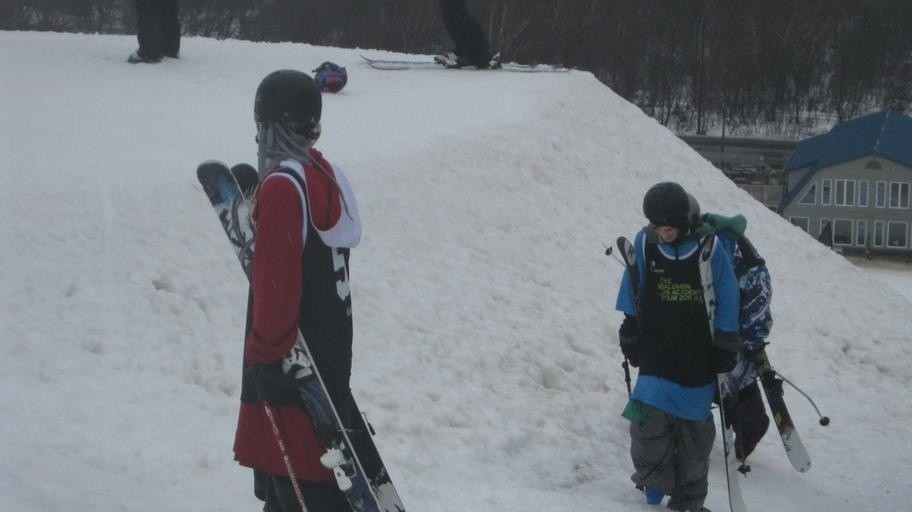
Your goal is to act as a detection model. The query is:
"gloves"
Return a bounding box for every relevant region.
[238,358,289,403]
[619,318,644,367]
[710,348,740,375]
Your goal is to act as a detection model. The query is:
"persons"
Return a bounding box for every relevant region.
[231,66,368,511]
[613,182,741,511]
[432,2,493,70]
[124,1,184,65]
[687,193,777,474]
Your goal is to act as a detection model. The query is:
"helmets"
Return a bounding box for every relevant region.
[641,178,689,227]
[313,60,350,94]
[683,192,701,224]
[252,69,325,130]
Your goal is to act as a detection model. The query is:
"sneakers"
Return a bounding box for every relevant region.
[127,49,162,65]
[642,485,666,506]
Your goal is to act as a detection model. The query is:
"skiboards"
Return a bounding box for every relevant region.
[197,160,405,512]
[757,348,812,473]
[617,234,744,512]
[361,55,577,73]
